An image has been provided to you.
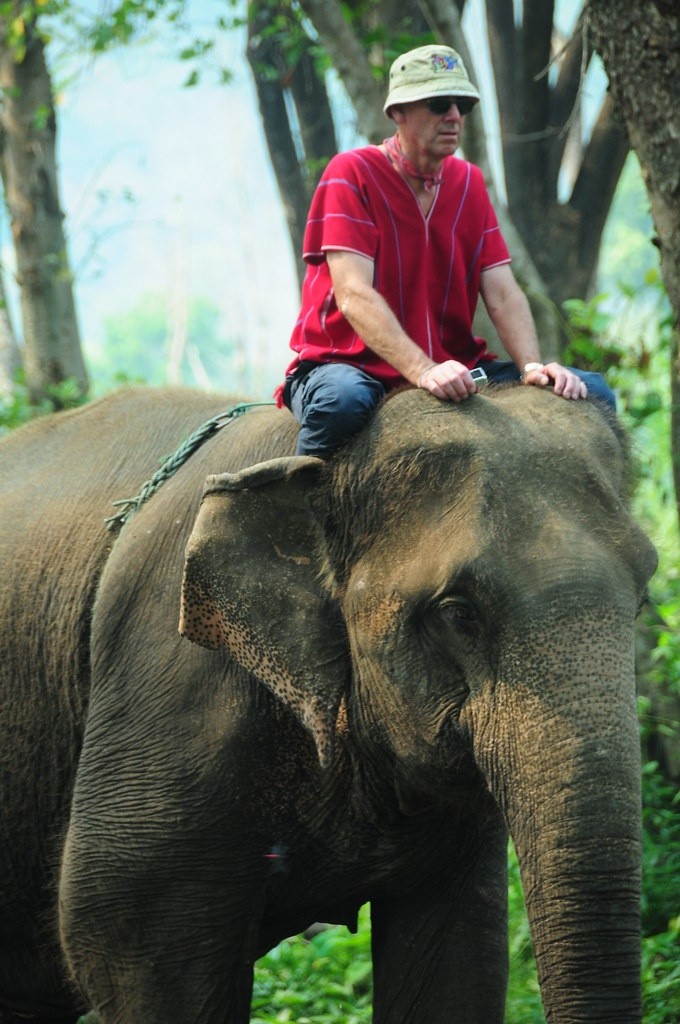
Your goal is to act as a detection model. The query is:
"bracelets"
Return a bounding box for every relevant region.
[518,361,544,384]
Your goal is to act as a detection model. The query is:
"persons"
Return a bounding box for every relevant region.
[273,42,618,462]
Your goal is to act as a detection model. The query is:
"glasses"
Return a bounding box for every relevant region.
[423,96,474,116]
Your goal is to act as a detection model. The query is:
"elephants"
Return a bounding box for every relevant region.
[0,369,660,1024]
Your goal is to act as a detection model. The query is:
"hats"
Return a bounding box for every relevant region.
[382,46,481,118]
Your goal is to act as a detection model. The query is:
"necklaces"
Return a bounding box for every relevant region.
[383,130,448,195]
